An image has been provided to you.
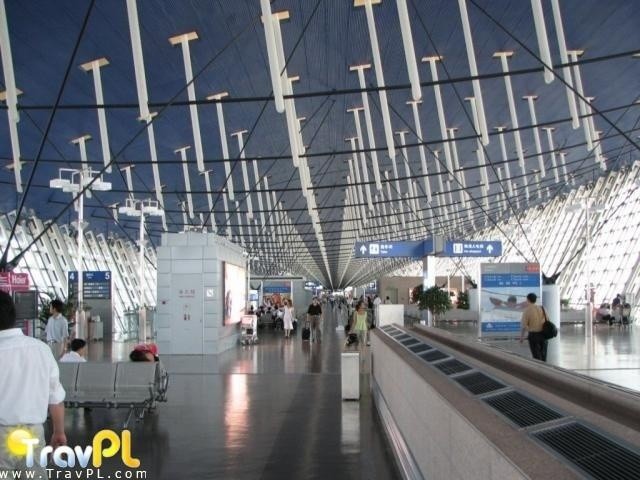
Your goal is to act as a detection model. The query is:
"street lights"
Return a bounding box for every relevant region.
[503,222,532,261]
[118,198,164,342]
[563,194,611,341]
[51,168,106,339]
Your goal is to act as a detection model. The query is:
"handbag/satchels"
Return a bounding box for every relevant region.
[543,320,557,340]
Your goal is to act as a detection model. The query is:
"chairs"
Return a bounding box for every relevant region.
[56,360,169,428]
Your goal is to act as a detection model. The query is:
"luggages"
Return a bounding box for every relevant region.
[301,321,311,340]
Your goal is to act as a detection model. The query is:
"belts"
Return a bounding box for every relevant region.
[49,340,57,343]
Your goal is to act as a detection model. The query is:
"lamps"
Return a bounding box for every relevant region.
[1,0,295,279]
[255,0,609,279]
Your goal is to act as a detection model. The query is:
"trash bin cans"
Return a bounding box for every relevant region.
[340,353,360,401]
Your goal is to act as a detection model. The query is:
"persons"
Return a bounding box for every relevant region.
[130,349,156,361]
[520,292,552,361]
[612,293,622,310]
[622,303,631,324]
[489,295,529,312]
[58,339,86,362]
[0,290,67,480]
[599,303,615,325]
[44,299,70,360]
[250,292,393,353]
